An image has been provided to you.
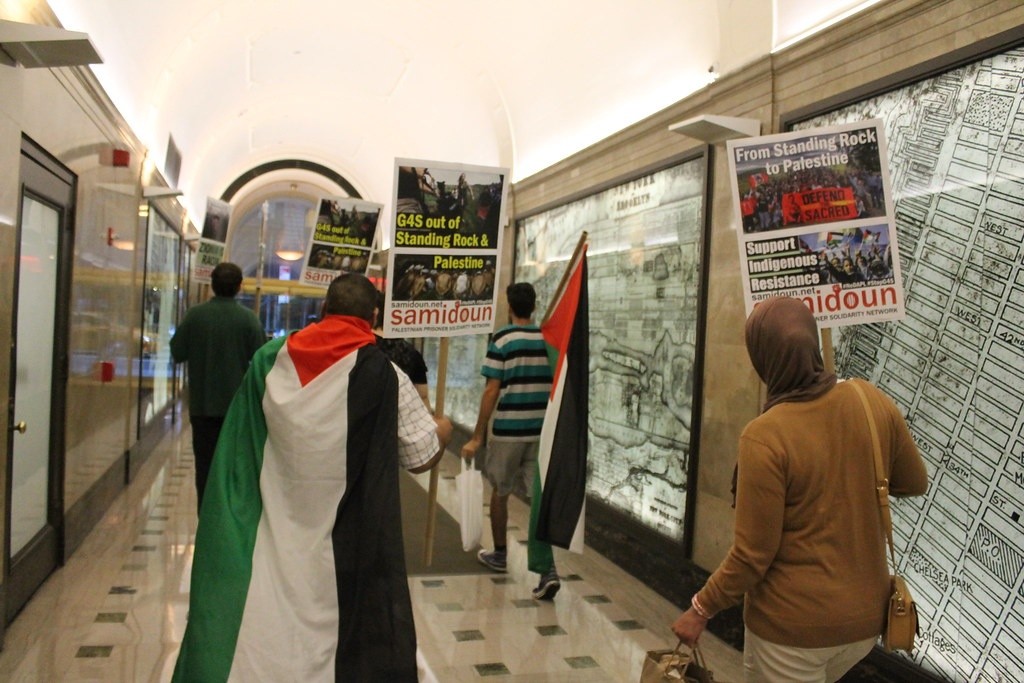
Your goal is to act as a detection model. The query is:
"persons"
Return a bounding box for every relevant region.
[394,259,496,303]
[670,295,929,683]
[396,165,505,249]
[371,290,434,414]
[169,261,268,519]
[461,281,560,601]
[804,240,894,285]
[738,162,884,234]
[203,275,452,683]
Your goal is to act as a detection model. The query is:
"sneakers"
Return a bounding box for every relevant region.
[532,572,560,599]
[477,549,508,571]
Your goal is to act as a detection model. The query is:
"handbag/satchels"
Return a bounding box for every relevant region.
[455,456,483,552]
[884,576,921,654]
[640,640,716,683]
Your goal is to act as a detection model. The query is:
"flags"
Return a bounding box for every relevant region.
[527,241,590,574]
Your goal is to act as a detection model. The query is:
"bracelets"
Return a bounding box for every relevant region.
[691,592,712,619]
